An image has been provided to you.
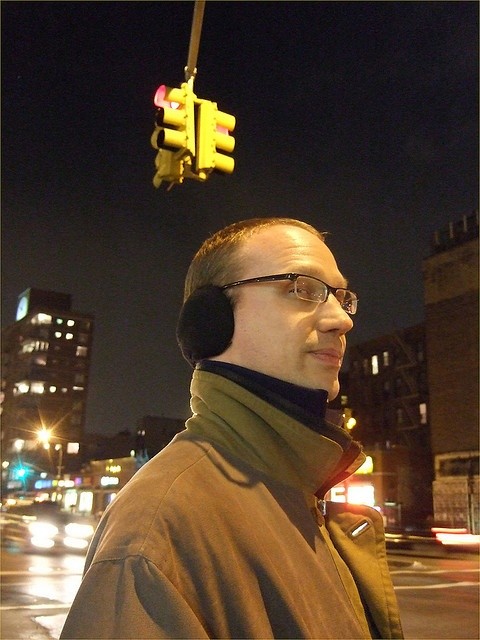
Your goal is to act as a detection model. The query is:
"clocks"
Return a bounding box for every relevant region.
[15,296,29,322]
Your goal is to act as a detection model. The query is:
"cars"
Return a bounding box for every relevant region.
[1,492,95,556]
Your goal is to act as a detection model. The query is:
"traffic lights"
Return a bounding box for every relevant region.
[150,156,181,194]
[154,84,196,157]
[196,105,237,173]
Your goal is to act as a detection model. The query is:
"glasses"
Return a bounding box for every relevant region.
[220,274,360,316]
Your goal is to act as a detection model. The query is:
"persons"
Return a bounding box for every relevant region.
[60,216,404,640]
